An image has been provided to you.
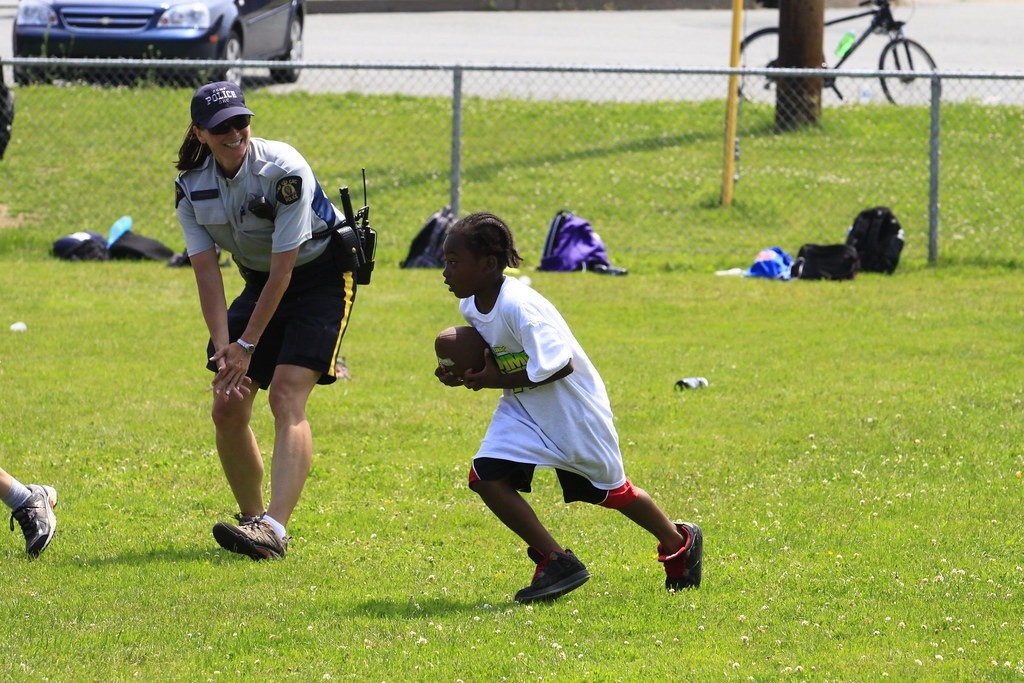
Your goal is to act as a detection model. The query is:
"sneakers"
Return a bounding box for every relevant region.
[658,520,702,594]
[513,545,592,604]
[9,483,59,562]
[212,513,288,561]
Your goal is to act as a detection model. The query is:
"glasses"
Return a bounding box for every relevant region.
[203,115,250,135]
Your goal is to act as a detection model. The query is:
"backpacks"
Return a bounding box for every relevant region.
[540,210,609,272]
[401,205,454,270]
[847,206,905,275]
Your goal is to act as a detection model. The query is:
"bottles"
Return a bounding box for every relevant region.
[835,29,856,57]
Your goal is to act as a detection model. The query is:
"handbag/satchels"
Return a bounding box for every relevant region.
[796,244,859,281]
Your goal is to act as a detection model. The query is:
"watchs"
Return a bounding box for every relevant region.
[236,339,255,354]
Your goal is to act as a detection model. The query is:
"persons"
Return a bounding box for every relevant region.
[0,56,57,561]
[433,210,703,606]
[172,79,377,561]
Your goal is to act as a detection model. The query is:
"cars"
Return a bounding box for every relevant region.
[11,0,306,93]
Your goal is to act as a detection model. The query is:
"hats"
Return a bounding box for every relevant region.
[191,80,255,130]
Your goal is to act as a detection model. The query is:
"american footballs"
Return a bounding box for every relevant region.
[434,326,487,375]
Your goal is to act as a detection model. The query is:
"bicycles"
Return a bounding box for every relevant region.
[735,0,949,110]
